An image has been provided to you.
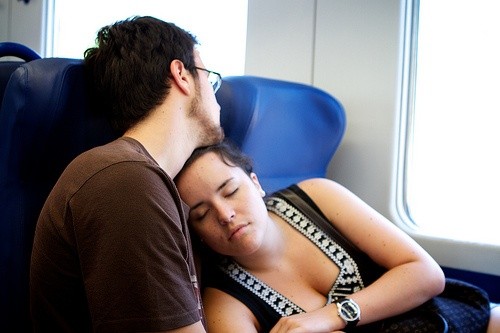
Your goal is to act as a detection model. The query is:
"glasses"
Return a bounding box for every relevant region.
[194,66,222,94]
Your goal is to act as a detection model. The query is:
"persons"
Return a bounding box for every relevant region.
[28,17,225,333]
[176,146,500,333]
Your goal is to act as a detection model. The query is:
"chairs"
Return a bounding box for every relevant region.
[1,41,346,333]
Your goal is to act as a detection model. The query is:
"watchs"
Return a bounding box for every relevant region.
[335,298,360,332]
